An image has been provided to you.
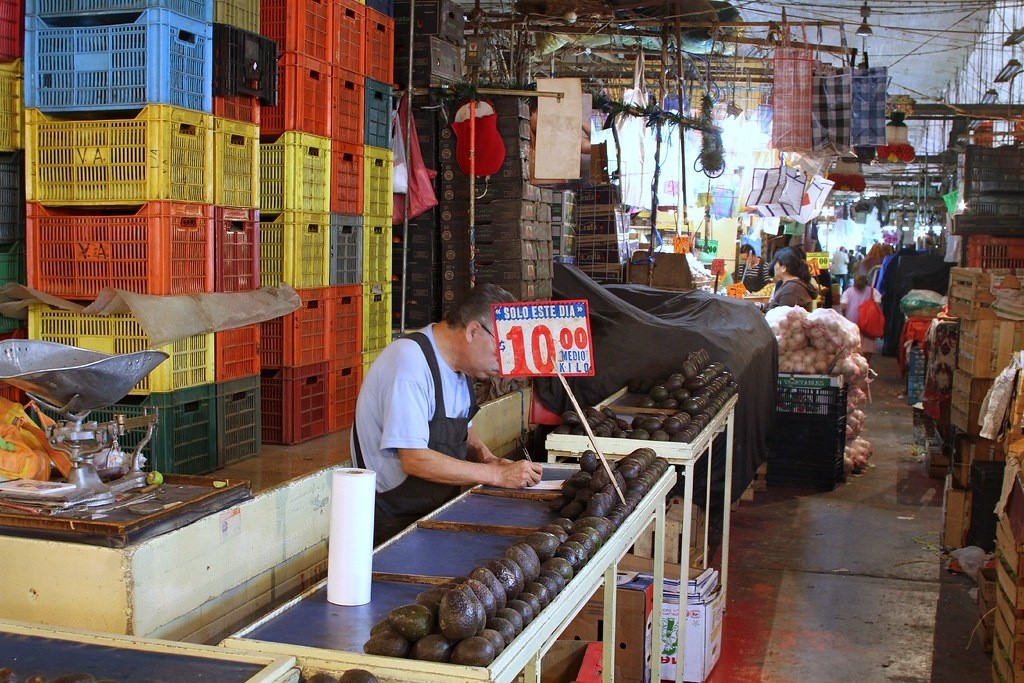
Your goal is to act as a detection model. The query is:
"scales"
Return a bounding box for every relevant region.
[0,338,171,505]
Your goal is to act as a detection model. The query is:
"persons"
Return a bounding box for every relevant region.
[833,244,867,293]
[754,245,817,313]
[738,244,772,294]
[840,274,882,370]
[528,94,592,184]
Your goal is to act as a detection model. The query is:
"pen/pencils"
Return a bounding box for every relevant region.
[518,438,533,463]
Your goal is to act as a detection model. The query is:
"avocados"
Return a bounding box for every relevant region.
[553,349,740,444]
[363,447,670,669]
[305,669,379,683]
[0,666,96,683]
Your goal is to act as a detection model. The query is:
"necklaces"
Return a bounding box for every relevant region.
[349,282,542,552]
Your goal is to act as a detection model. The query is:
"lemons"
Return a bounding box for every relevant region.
[146,471,163,485]
[213,481,227,488]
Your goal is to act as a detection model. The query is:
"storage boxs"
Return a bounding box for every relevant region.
[393,0,624,328]
[541,553,724,683]
[0,0,392,476]
[765,372,848,490]
[907,314,932,406]
[923,143,1024,683]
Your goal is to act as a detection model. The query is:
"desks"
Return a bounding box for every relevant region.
[0,620,302,683]
[545,384,738,683]
[218,463,678,683]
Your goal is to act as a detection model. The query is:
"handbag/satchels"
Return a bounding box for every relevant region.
[772,20,888,159]
[746,154,835,224]
[859,287,885,337]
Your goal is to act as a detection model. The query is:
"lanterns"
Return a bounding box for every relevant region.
[827,111,915,191]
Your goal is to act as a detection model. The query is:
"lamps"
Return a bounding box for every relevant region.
[854,0,873,36]
